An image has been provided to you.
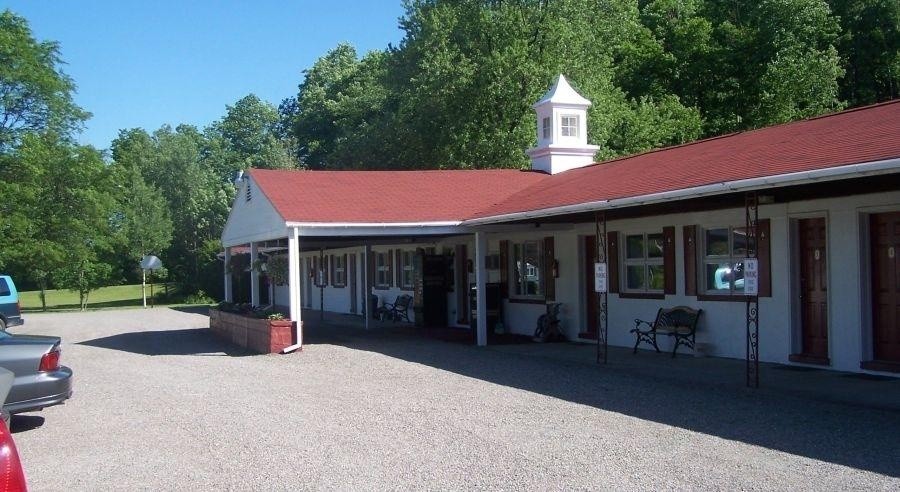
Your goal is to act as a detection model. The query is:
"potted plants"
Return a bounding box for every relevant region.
[209,301,302,354]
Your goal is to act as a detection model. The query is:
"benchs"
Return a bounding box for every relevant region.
[631,306,702,358]
[378,295,413,323]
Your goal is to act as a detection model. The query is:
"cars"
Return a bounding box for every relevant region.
[0,275,73,492]
[713,266,745,292]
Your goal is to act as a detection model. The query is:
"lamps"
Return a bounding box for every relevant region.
[552,259,558,278]
[235,169,249,183]
